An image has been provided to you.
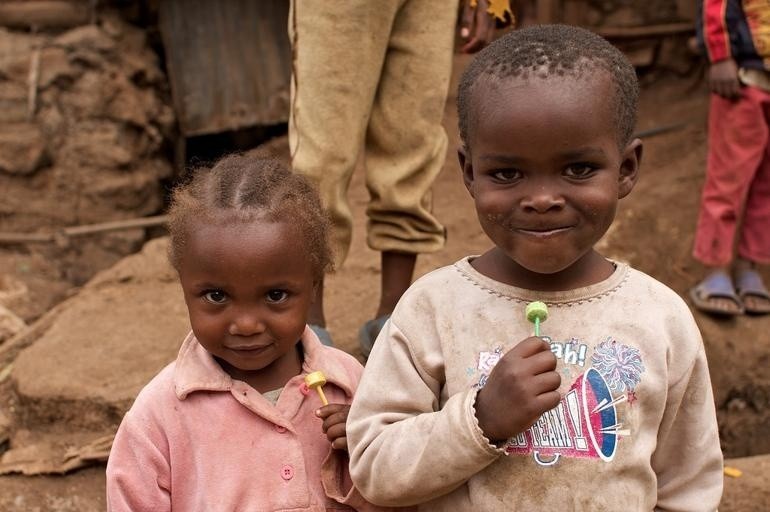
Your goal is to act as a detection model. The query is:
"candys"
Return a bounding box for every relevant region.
[526,302,549,336]
[304,370,332,405]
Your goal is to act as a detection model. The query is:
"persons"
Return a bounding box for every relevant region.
[283,0,461,359]
[345,22,725,512]
[688,0,770,318]
[105,150,417,512]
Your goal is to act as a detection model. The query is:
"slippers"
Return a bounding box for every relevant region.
[690,269,770,317]
[359,314,391,357]
